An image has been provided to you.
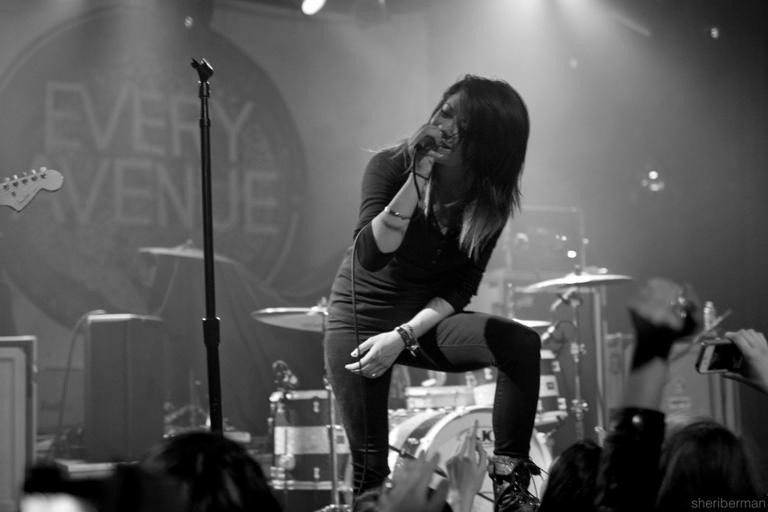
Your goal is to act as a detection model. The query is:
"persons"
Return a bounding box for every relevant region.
[535,439,600,512]
[140,431,280,512]
[272,361,299,426]
[610,281,768,511]
[324,74,540,510]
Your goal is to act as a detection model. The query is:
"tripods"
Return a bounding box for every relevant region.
[164,366,208,427]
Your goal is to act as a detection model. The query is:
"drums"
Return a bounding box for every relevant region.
[404,384,477,409]
[380,406,551,512]
[267,390,350,481]
[465,349,567,427]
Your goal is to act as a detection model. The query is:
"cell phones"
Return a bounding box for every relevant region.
[695,339,744,374]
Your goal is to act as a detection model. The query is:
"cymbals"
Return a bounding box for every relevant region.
[511,318,552,328]
[524,270,633,291]
[255,307,330,332]
[142,240,228,260]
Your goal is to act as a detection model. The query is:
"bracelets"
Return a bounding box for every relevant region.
[395,327,419,357]
[404,323,417,344]
[383,207,412,220]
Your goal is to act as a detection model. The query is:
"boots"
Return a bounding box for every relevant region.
[487,455,545,510]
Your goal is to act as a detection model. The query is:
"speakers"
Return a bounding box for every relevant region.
[83,313,165,464]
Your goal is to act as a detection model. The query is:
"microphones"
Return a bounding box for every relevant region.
[414,129,445,150]
